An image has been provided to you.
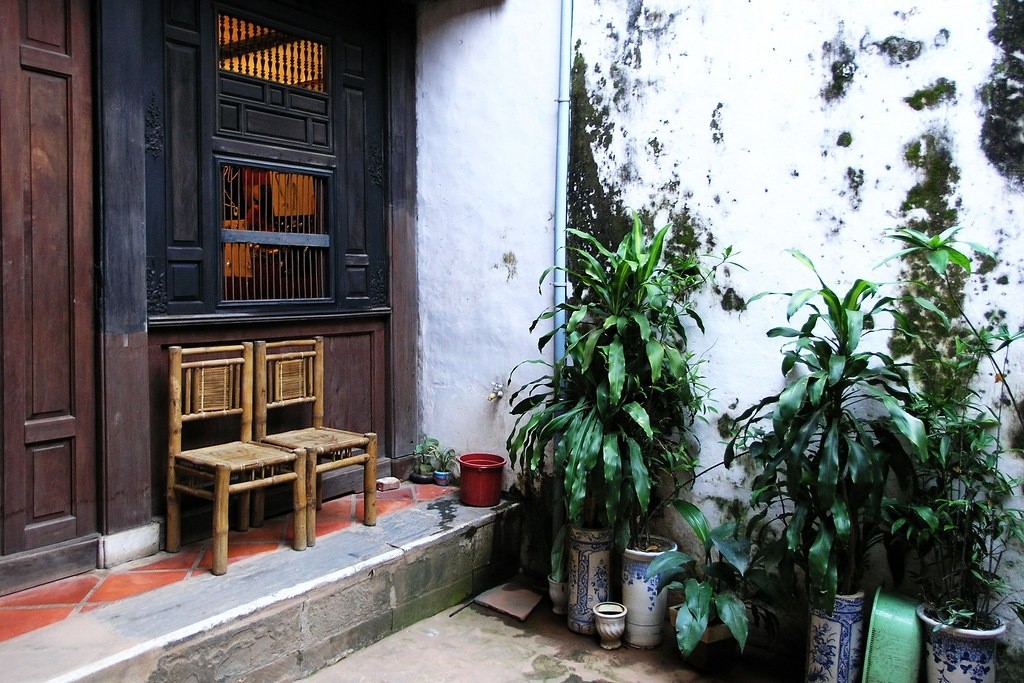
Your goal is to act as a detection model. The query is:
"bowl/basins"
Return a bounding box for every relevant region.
[862,585,923,683]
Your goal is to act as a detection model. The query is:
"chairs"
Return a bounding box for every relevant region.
[168,343,306,575]
[255,337,377,546]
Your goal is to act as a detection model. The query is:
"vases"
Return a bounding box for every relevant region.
[594,601,628,649]
[548,574,566,615]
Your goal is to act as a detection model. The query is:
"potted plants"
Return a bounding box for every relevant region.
[501,206,1023,683]
[411,433,459,486]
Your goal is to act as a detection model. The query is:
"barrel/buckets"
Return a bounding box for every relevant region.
[456,453,506,507]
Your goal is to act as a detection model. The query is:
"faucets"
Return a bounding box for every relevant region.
[487,381,504,402]
